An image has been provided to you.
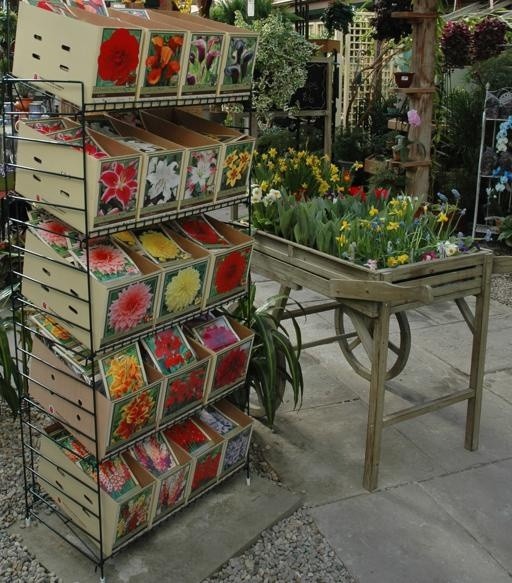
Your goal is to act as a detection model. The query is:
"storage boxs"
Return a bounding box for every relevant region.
[12,1,259,556]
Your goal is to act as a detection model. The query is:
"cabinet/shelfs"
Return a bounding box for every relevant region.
[265,51,336,159]
[465,80,512,246]
[1,78,256,582]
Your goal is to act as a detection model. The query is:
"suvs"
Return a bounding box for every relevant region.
[0,100,49,153]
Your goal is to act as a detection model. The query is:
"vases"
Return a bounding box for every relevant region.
[393,70,415,87]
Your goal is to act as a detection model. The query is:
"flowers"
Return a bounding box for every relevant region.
[406,108,422,142]
[251,145,473,270]
